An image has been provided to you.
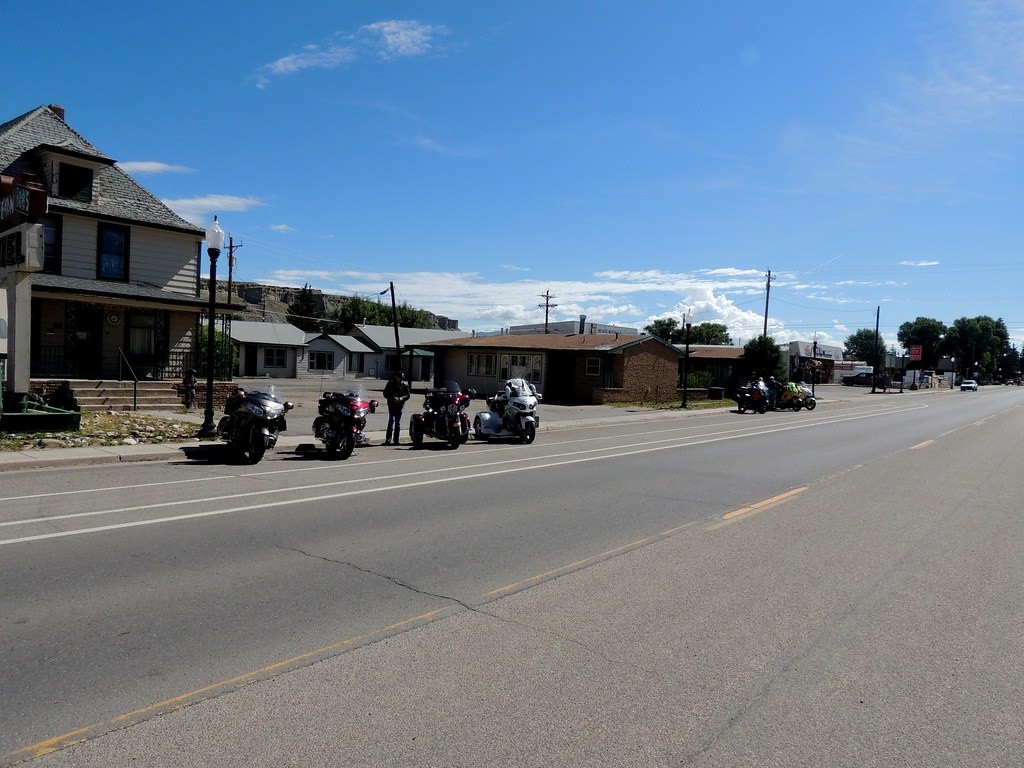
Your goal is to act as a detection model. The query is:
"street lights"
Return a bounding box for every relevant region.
[195,215,224,436]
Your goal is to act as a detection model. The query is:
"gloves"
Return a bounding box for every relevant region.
[399,397,403,402]
[393,397,399,404]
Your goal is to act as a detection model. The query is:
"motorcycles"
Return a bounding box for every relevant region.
[409,380,476,450]
[217,384,294,465]
[736,381,817,414]
[312,384,379,460]
[472,378,543,444]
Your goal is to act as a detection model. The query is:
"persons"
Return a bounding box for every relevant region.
[183,370,197,409]
[383,371,410,446]
[758,376,782,411]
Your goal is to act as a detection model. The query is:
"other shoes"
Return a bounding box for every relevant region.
[394,441,400,446]
[385,439,391,443]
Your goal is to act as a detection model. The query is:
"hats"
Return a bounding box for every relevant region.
[396,371,405,378]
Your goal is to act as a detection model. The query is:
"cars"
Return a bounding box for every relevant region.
[842,372,873,387]
[960,380,978,391]
